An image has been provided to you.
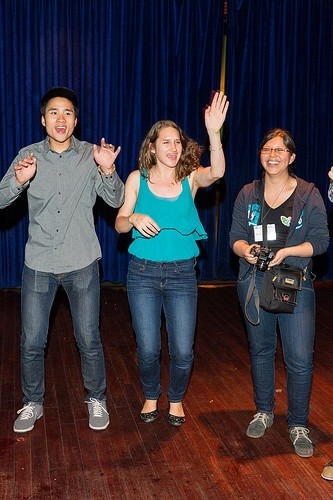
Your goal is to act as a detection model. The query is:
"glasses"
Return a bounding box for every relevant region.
[260,147,290,155]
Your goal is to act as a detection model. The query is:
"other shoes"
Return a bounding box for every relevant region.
[167,400,186,427]
[321,460,333,480]
[139,397,159,423]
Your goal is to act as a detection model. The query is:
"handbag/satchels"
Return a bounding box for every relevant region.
[260,263,306,314]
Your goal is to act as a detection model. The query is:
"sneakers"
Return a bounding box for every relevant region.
[13,402,43,432]
[289,426,313,457]
[246,412,273,438]
[84,397,110,430]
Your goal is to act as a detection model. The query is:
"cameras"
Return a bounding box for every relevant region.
[251,247,276,272]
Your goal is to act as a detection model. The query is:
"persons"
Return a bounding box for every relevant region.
[114,92,229,426]
[230,130,329,458]
[0,86,127,432]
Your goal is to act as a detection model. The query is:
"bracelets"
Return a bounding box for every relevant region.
[97,164,117,176]
[208,144,222,152]
